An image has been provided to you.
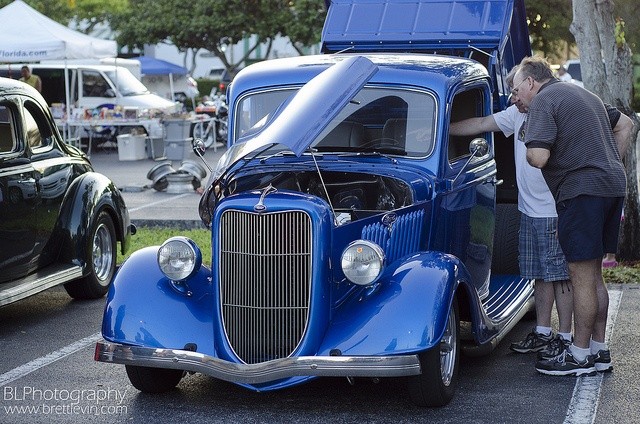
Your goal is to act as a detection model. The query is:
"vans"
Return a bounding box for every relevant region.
[0,64,184,146]
[141,74,199,102]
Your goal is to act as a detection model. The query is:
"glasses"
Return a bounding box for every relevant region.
[511,76,529,96]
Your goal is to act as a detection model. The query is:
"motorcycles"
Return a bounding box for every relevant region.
[186,84,228,149]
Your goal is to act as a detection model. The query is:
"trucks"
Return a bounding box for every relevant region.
[95,1,536,408]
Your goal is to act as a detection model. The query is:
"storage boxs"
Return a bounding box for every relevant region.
[165,118,190,140]
[116,133,146,161]
[165,138,192,159]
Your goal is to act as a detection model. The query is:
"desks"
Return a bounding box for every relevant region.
[69,119,165,162]
[54,119,80,146]
[164,117,217,159]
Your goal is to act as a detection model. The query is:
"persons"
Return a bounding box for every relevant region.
[415,64,574,359]
[17,66,42,95]
[512,56,634,379]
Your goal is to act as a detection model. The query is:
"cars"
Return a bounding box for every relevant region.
[0,76,136,307]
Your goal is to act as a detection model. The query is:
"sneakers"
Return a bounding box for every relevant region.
[535,347,597,377]
[592,349,612,372]
[537,334,573,359]
[510,328,554,354]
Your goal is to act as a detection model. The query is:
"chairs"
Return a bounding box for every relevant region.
[380,118,459,159]
[320,121,365,150]
